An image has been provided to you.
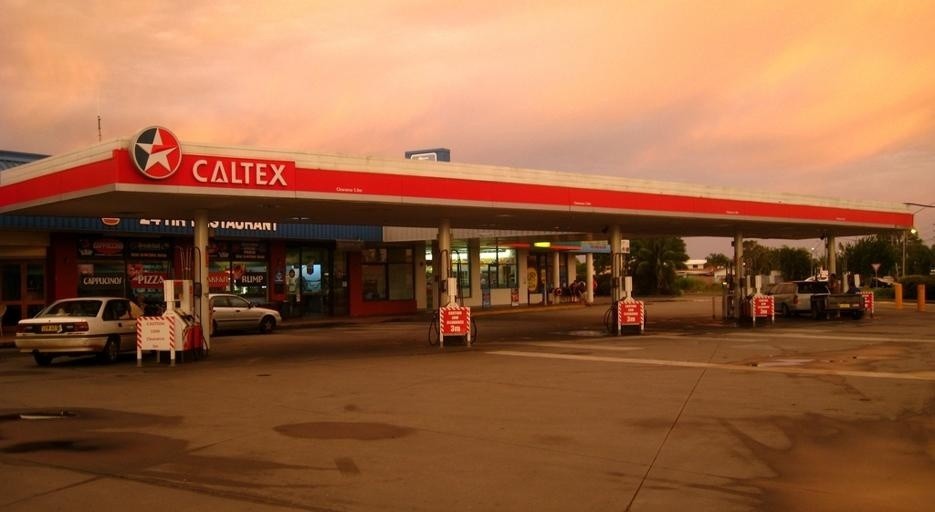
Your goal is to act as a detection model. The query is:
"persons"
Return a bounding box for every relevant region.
[566,275,598,303]
[826,272,841,320]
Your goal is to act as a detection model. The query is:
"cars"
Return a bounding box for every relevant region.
[766,281,831,317]
[14,296,145,362]
[207,293,282,336]
